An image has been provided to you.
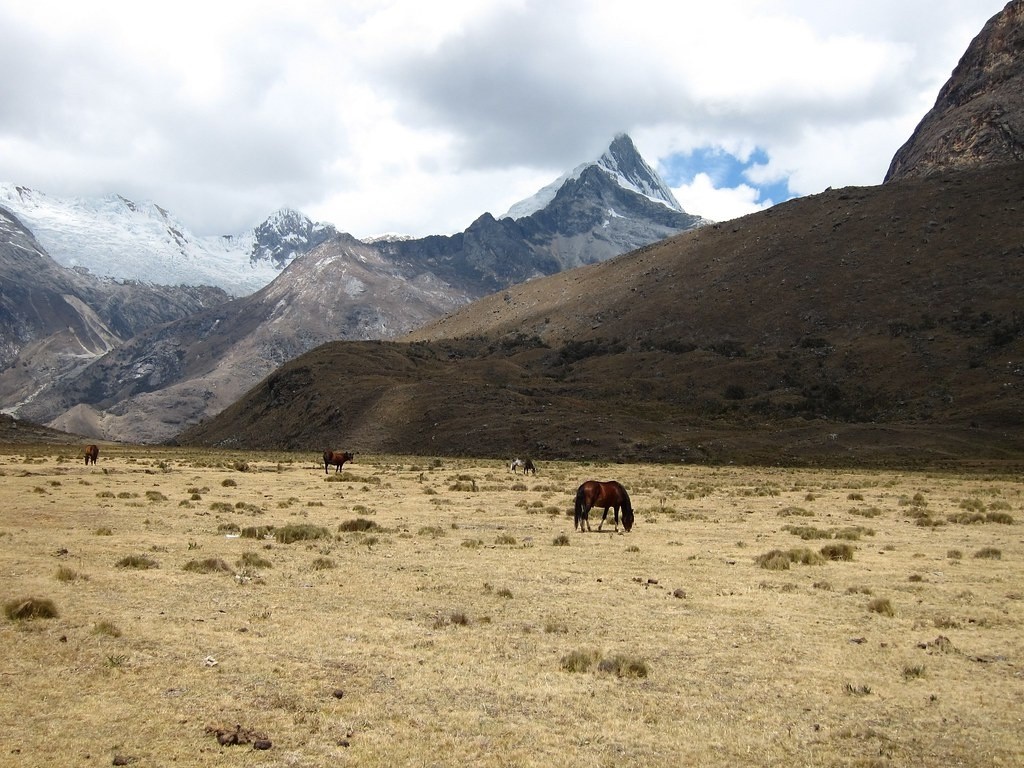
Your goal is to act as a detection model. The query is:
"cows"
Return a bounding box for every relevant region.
[323,450,354,474]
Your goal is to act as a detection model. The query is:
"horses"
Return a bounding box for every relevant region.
[84,445,99,466]
[511,457,536,475]
[574,481,634,533]
